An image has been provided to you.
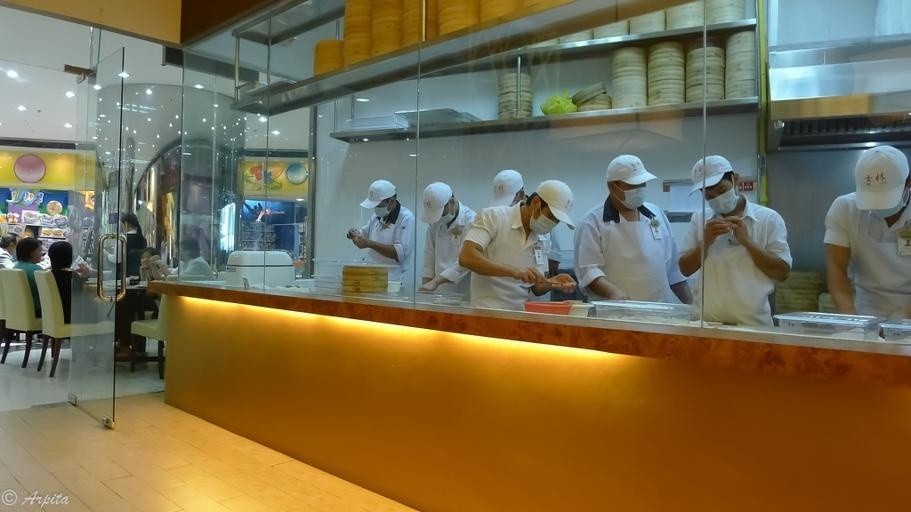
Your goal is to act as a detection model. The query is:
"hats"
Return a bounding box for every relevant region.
[357,179,397,210]
[492,168,524,208]
[604,153,658,187]
[853,145,911,211]
[419,180,453,226]
[685,153,734,199]
[534,178,576,233]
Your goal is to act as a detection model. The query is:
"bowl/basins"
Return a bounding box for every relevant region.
[389,281,401,295]
[410,290,467,306]
[524,301,595,319]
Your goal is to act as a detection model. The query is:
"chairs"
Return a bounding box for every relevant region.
[0,264,21,347]
[130,274,204,379]
[34,271,116,377]
[0,269,54,367]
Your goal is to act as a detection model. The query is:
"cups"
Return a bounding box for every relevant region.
[88,271,139,286]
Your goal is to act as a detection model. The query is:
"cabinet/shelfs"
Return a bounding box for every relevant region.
[329,17,761,143]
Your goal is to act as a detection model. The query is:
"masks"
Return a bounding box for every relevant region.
[704,176,741,217]
[437,203,457,223]
[528,209,557,236]
[613,182,651,209]
[865,190,908,221]
[372,198,394,218]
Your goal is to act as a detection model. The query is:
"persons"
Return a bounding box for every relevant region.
[490,170,530,209]
[458,180,577,312]
[49,241,104,324]
[676,155,794,327]
[823,145,911,324]
[13,237,46,318]
[76,211,214,370]
[1,235,19,269]
[243,202,286,225]
[346,180,416,274]
[418,182,477,293]
[573,154,695,304]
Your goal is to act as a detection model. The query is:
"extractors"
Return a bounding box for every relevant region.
[768,33,910,152]
